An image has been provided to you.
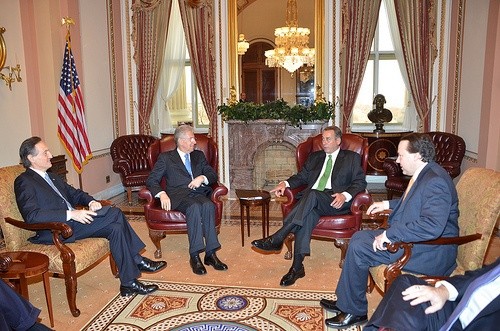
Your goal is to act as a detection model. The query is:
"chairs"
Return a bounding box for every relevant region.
[383,132,466,200]
[138,134,228,259]
[0,165,118,317]
[366,167,500,298]
[110,134,156,206]
[275,134,369,268]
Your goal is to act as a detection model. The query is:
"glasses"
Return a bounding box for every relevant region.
[181,137,197,142]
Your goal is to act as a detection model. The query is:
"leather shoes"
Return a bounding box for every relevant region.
[204,255,228,271]
[251,236,283,255]
[190,255,206,274]
[136,256,167,274]
[120,278,159,297]
[325,311,367,328]
[320,299,341,312]
[280,264,305,286]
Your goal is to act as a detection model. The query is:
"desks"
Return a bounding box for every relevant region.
[352,132,412,176]
[0,251,54,328]
[235,189,271,247]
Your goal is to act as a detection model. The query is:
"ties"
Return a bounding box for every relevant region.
[438,264,500,331]
[184,154,192,178]
[403,178,415,200]
[45,173,72,210]
[317,155,332,191]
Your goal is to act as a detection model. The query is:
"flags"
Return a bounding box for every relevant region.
[56,32,92,174]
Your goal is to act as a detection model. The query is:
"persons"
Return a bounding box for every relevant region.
[250,127,368,287]
[240,92,247,103]
[320,132,460,329]
[145,125,228,275]
[367,94,393,124]
[0,278,55,331]
[362,254,500,331]
[13,136,167,296]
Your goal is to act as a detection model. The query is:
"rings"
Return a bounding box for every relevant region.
[417,287,420,290]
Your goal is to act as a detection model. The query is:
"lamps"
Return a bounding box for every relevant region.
[265,0,316,79]
[237,12,249,56]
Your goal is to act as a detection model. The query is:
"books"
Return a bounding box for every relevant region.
[235,189,262,199]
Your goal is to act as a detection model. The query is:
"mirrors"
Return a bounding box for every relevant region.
[226,0,325,102]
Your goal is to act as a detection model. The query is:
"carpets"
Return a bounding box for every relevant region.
[80,279,362,331]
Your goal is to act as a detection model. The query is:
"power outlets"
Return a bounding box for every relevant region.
[106,176,110,183]
